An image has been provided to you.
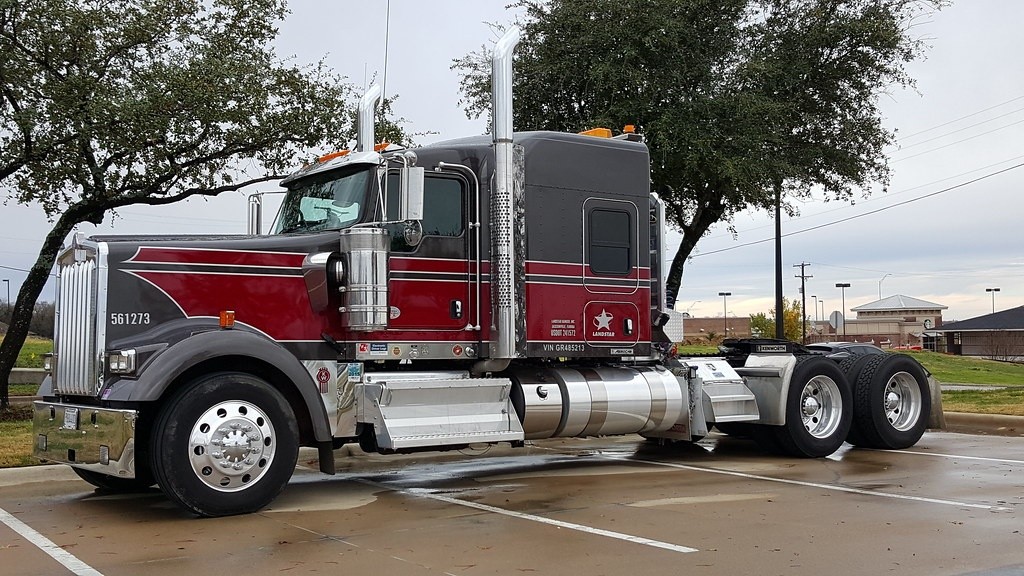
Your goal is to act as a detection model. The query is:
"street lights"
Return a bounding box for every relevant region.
[835,283,850,341]
[986,288,1000,314]
[878,274,892,300]
[820,301,824,322]
[811,295,818,321]
[719,292,732,337]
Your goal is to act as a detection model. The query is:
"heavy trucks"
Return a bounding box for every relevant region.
[29,23,931,516]
